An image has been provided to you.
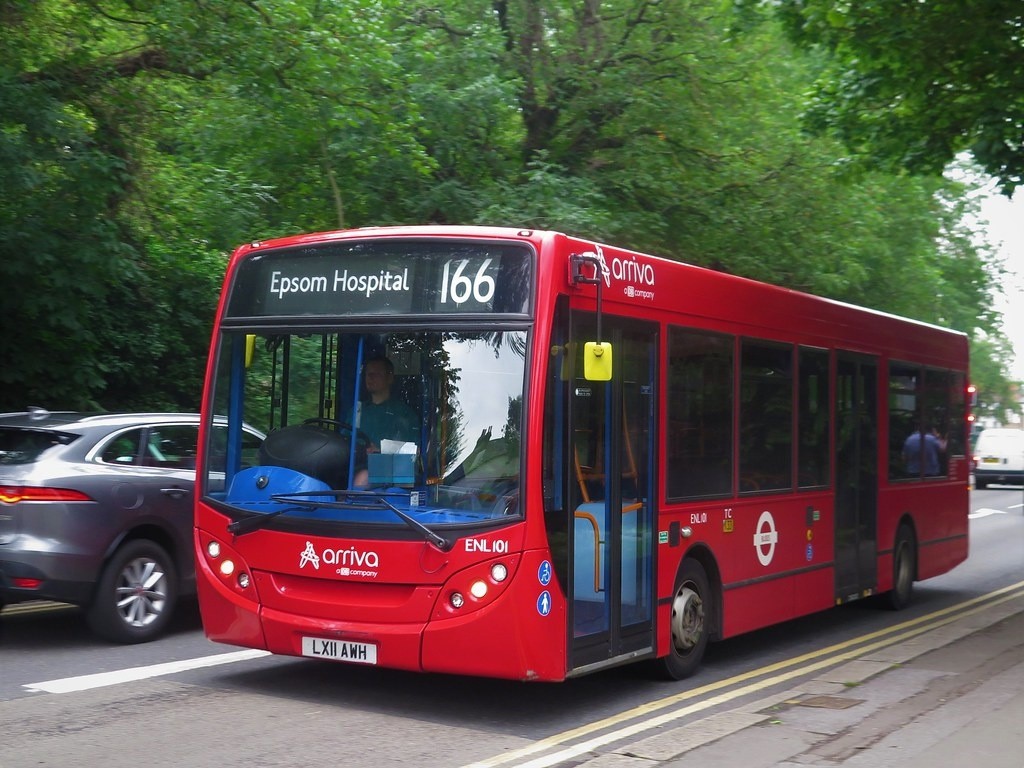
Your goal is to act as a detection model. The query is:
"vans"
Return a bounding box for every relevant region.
[972,428,1024,490]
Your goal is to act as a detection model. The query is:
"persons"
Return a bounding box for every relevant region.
[901,419,949,477]
[339,355,422,490]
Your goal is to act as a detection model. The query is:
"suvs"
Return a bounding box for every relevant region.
[0,404,267,649]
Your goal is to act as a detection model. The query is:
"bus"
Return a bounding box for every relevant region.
[193,224,973,685]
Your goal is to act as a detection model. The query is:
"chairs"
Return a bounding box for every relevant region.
[741,471,820,490]
[148,429,176,460]
